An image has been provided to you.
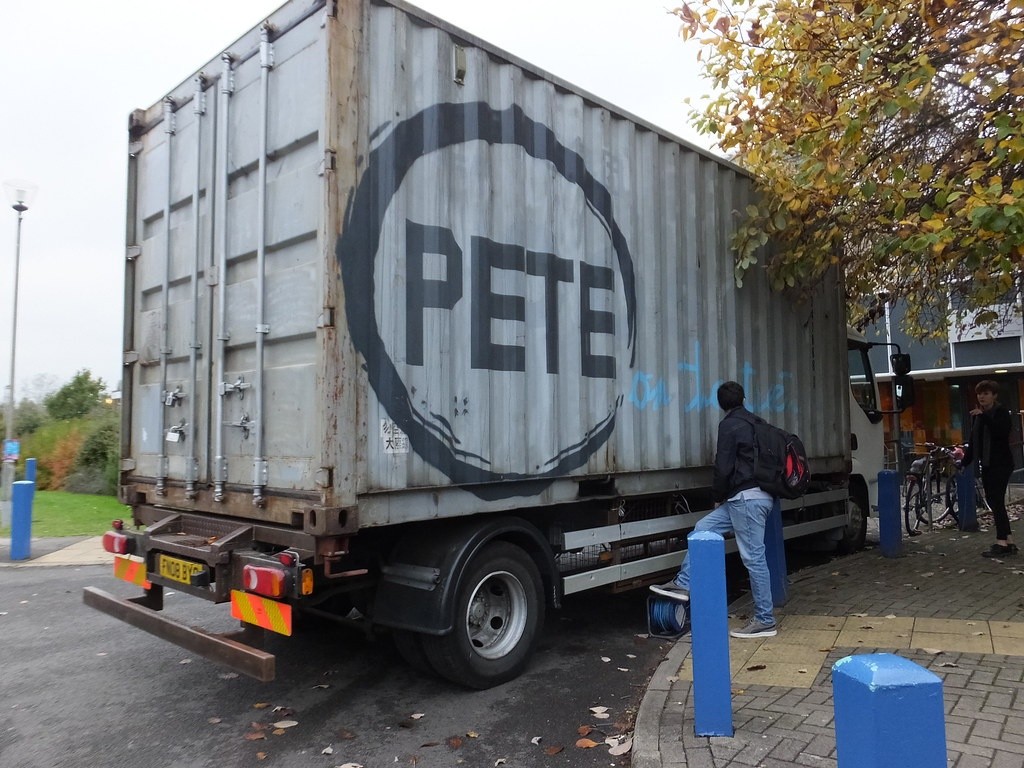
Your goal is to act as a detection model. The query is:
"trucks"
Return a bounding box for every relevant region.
[84,0,914,691]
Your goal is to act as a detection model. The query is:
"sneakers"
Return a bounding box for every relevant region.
[981,544,1011,558]
[1008,543,1018,555]
[729,616,777,638]
[649,576,689,601]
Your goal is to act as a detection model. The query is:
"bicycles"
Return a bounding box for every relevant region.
[902,441,964,536]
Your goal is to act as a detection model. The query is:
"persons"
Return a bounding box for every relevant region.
[649,381,777,638]
[956,381,1017,558]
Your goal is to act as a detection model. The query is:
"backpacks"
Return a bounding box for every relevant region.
[727,413,810,499]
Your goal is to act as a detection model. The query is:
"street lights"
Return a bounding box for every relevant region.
[1,199,29,500]
